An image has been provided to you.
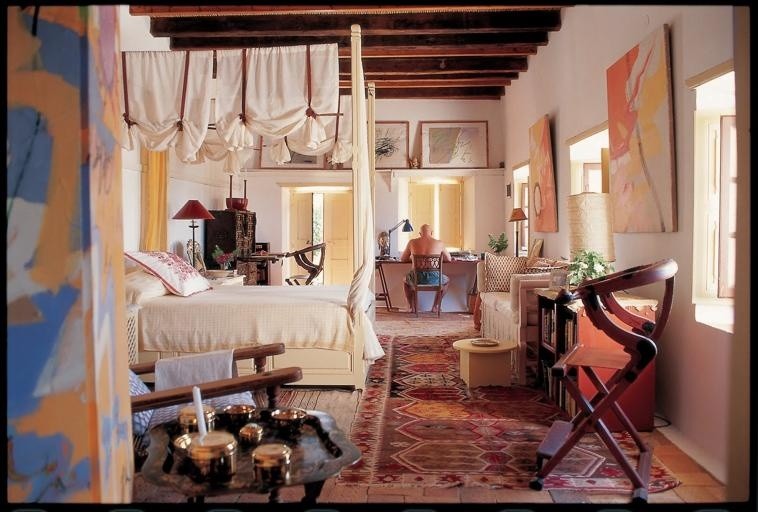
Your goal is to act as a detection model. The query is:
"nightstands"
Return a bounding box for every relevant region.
[202,275,246,286]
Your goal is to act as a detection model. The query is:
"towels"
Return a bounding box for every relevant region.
[155,347,238,391]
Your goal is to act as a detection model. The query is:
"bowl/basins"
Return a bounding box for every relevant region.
[223,405,256,428]
[270,408,308,436]
[171,431,201,458]
[225,197,248,210]
[205,270,231,277]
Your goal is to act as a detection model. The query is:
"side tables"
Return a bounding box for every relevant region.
[143,411,362,502]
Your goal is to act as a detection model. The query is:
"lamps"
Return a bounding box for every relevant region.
[378,219,414,260]
[509,208,528,256]
[172,200,215,266]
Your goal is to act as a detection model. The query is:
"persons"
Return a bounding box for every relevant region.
[398,224,452,313]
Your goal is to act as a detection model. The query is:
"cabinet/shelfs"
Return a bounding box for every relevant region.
[536,288,658,433]
[204,209,285,286]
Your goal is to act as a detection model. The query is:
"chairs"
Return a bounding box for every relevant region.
[530,259,678,504]
[127,343,302,470]
[410,251,444,318]
[286,243,326,285]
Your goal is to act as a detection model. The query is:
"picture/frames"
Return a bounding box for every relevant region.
[339,121,409,169]
[420,120,489,169]
[259,134,327,170]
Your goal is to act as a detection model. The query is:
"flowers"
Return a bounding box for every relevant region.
[556,248,616,284]
[487,232,508,253]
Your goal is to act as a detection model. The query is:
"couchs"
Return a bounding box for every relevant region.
[476,252,574,387]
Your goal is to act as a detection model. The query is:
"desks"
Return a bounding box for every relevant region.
[374,258,482,313]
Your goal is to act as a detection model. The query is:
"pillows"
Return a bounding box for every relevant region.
[124,250,212,304]
[484,252,568,292]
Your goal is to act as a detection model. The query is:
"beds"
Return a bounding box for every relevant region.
[121,23,376,386]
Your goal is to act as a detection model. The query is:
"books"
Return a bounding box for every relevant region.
[554,377,577,418]
[563,318,577,353]
[539,359,553,400]
[541,302,557,350]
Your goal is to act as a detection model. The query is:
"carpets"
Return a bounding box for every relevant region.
[336,336,685,493]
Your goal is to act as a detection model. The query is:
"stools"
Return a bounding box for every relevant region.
[452,339,518,387]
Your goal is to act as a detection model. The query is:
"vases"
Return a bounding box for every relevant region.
[583,275,592,283]
[221,264,228,270]
[494,248,502,256]
[212,245,240,264]
